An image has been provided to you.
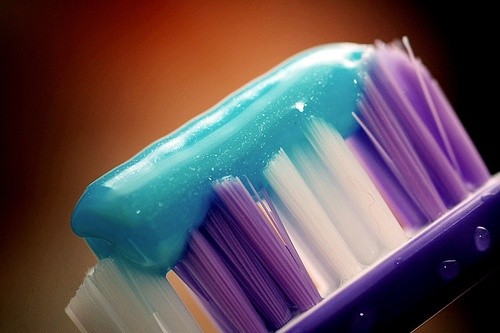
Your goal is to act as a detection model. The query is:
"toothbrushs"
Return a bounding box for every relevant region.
[65,35,500,332]
